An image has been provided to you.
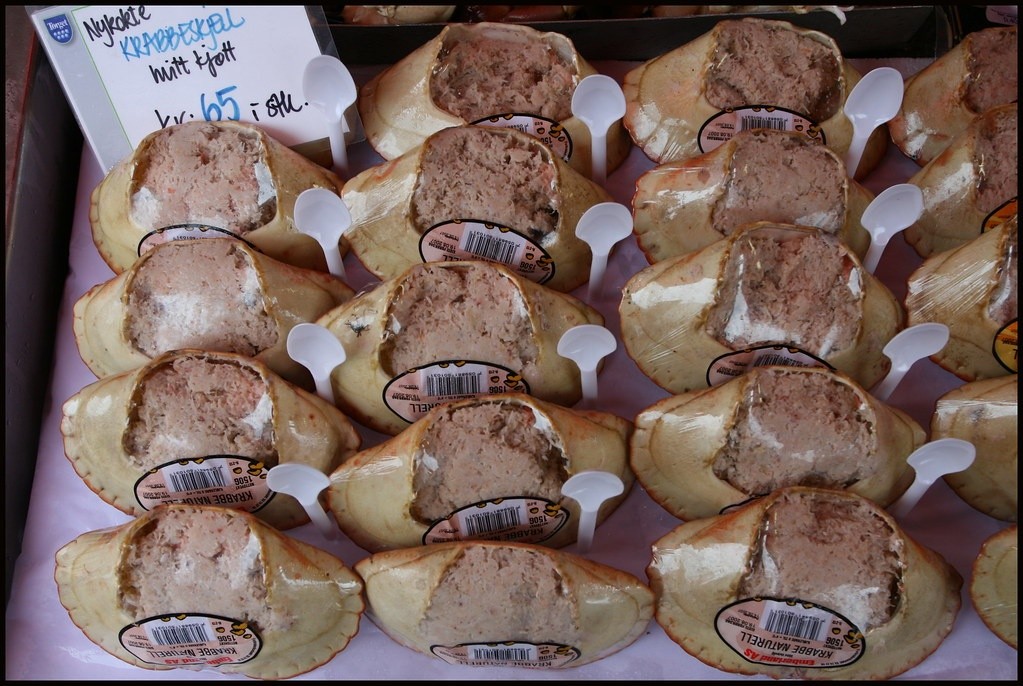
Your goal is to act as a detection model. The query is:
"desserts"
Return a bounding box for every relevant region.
[54,18,1019,683]
